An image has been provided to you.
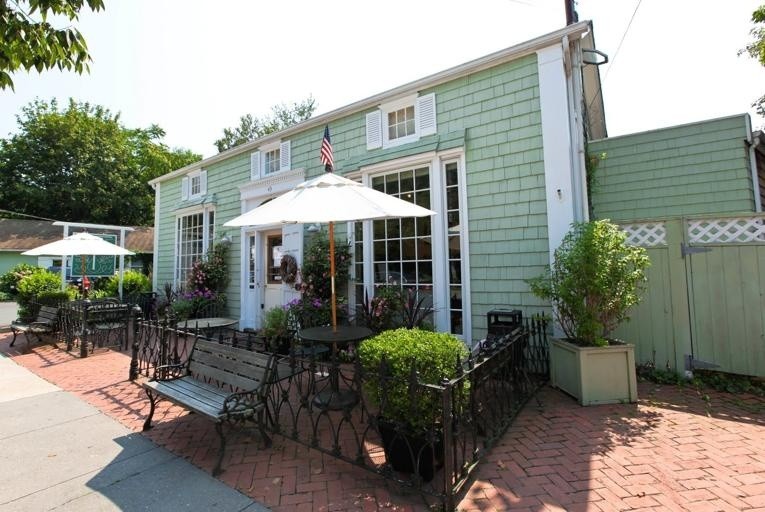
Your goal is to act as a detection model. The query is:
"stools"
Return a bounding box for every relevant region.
[239,327,268,350]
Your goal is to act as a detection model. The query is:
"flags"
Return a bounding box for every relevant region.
[319,125,333,169]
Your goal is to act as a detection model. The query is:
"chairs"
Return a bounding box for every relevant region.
[195,298,229,340]
[140,336,278,480]
[66,291,159,355]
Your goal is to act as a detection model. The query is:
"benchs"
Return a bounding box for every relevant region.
[9,305,61,354]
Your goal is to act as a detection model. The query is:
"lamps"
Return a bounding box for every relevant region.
[219,235,233,243]
[306,223,323,233]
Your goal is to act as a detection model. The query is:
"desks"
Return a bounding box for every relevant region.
[175,317,238,338]
[297,324,371,411]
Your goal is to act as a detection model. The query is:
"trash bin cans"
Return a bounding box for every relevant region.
[487,308,524,381]
[140,292,156,320]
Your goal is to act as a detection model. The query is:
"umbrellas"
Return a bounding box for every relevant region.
[222,168,438,395]
[20,231,138,296]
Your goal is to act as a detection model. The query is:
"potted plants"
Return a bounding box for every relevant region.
[354,325,471,482]
[526,215,653,407]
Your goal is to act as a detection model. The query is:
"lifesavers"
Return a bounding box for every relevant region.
[280,255,297,283]
